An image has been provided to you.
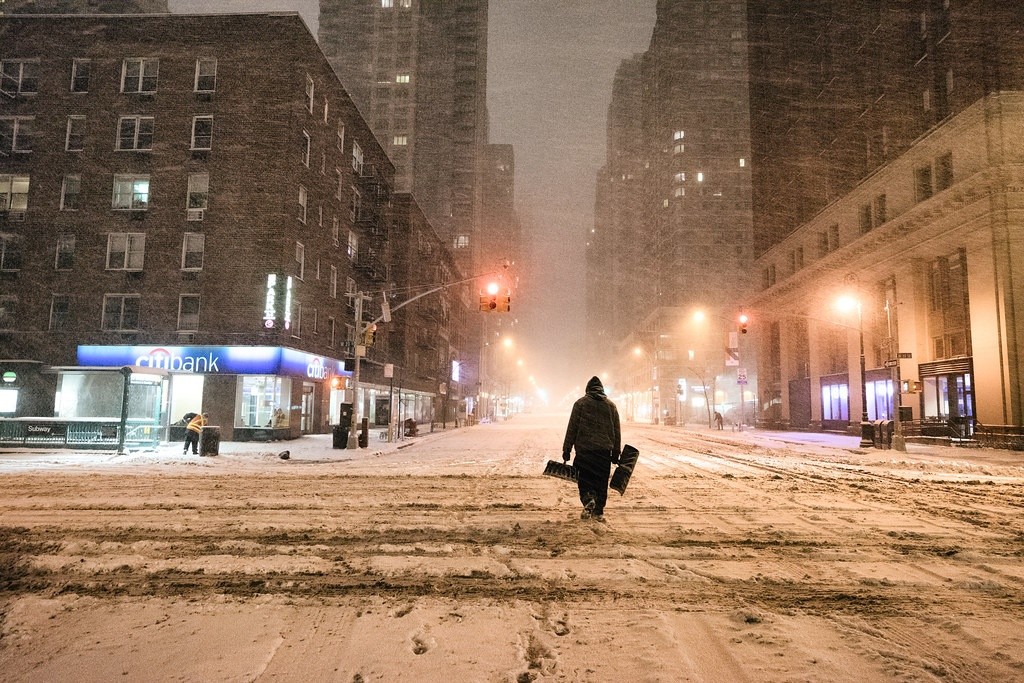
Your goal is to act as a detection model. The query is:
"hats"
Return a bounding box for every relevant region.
[586,377,604,394]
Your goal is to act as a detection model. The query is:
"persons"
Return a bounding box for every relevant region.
[714,412,725,430]
[269,409,285,426]
[562,376,622,525]
[182,412,208,455]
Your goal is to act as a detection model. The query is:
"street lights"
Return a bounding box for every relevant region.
[695,311,745,424]
[830,295,874,447]
[633,346,655,425]
[474,338,514,425]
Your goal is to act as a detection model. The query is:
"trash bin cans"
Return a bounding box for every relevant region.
[340,403,354,429]
[333,428,348,449]
[468,414,475,426]
[199,426,220,457]
[404,419,417,436]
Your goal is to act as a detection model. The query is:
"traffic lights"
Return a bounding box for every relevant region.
[738,313,748,334]
[479,283,511,313]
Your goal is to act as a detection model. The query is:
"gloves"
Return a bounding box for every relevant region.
[563,452,570,461]
[612,454,619,464]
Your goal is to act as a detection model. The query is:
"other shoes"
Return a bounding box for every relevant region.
[581,498,595,519]
[591,513,603,522]
[183,451,186,455]
[193,452,198,454]
